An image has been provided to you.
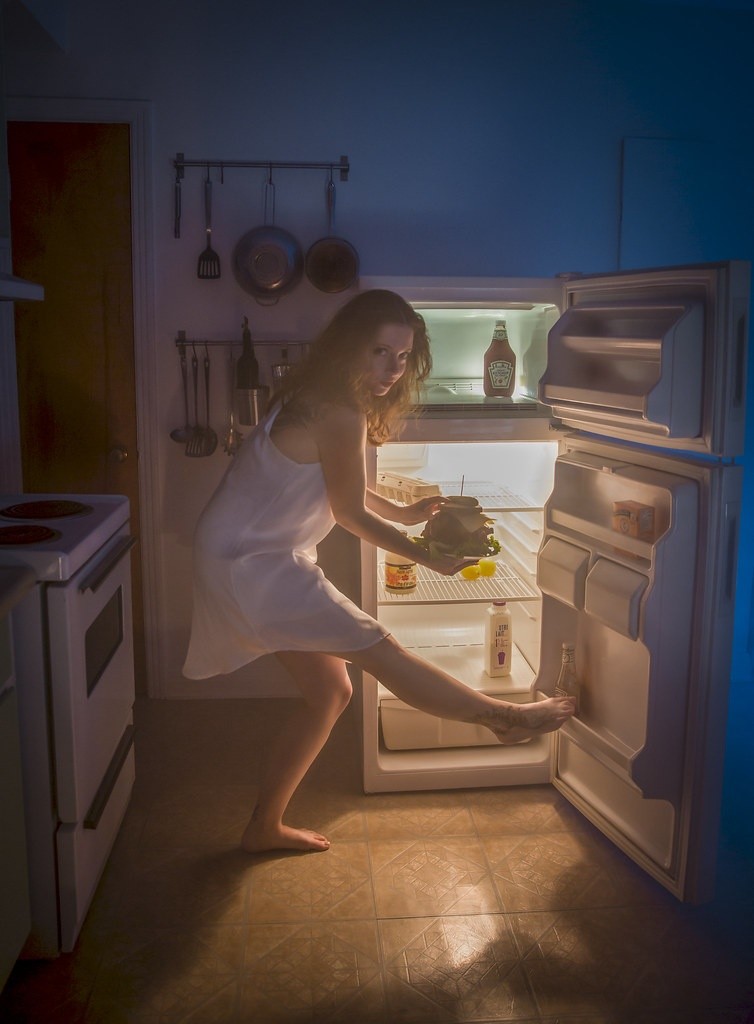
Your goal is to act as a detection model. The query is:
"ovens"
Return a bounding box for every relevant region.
[14,516,136,959]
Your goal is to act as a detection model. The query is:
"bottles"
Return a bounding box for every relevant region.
[554,640,581,719]
[385,530,417,594]
[484,602,513,677]
[484,320,517,398]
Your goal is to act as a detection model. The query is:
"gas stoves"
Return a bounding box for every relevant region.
[0,491,132,584]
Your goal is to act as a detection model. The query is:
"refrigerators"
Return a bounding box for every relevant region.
[338,259,735,905]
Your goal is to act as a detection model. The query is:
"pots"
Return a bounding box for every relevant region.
[305,181,360,294]
[234,181,304,307]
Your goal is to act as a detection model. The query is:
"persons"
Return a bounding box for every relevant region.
[181,288,576,851]
